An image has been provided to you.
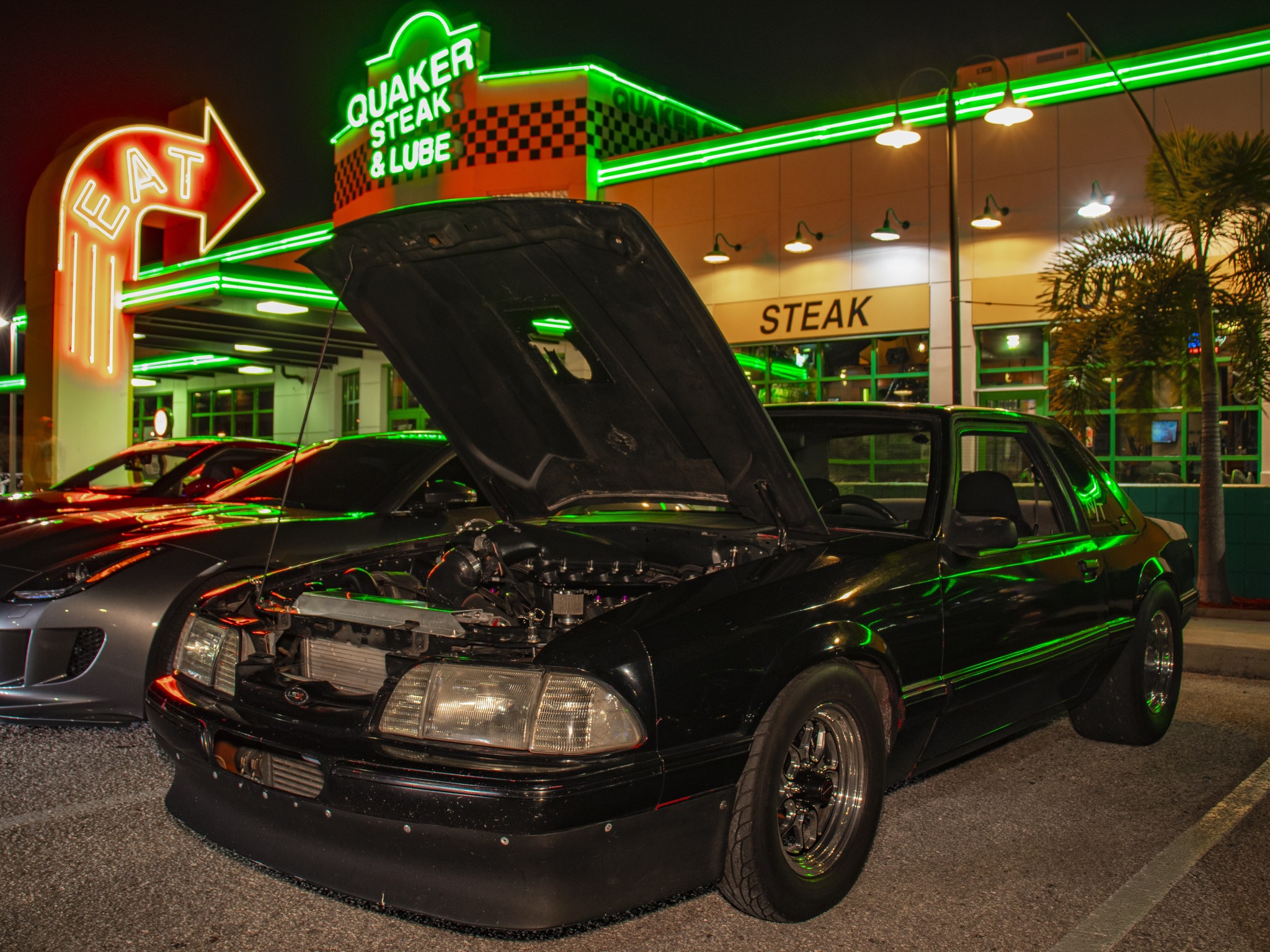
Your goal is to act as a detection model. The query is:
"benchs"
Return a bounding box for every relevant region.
[843,498,1103,536]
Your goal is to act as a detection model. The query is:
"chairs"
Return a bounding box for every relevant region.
[201,458,235,485]
[797,478,842,514]
[956,470,1033,538]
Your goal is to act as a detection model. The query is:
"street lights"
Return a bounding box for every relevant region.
[876,50,1033,408]
[1,311,27,474]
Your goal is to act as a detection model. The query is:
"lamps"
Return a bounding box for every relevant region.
[1077,180,1115,219]
[870,208,910,241]
[970,194,1010,230]
[703,233,741,264]
[784,221,823,253]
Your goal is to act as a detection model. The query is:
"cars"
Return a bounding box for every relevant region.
[0,436,448,729]
[142,196,1197,928]
